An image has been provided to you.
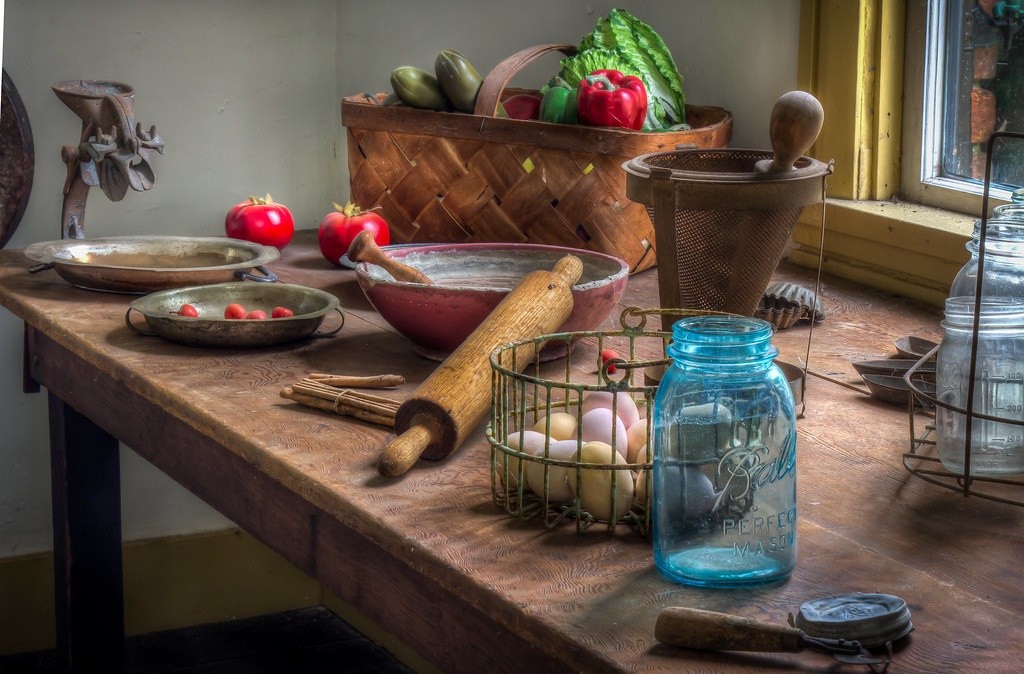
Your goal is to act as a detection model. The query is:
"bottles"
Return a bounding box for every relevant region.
[937,295,1024,474]
[653,316,798,591]
[949,187,1024,296]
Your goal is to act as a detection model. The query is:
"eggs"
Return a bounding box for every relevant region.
[495,387,751,517]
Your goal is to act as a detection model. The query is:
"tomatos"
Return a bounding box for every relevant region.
[225,192,295,251]
[318,200,390,267]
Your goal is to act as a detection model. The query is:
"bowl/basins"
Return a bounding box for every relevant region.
[356,241,629,362]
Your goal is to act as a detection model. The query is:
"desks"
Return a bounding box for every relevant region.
[0,217,1023,673]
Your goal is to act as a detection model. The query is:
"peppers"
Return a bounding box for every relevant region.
[502,69,648,131]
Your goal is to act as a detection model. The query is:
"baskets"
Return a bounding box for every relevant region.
[486,301,773,543]
[340,44,734,279]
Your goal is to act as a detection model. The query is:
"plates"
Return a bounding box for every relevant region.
[852,336,941,407]
[125,281,347,350]
[21,234,280,292]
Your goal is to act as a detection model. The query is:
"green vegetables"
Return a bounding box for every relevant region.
[536,8,687,131]
